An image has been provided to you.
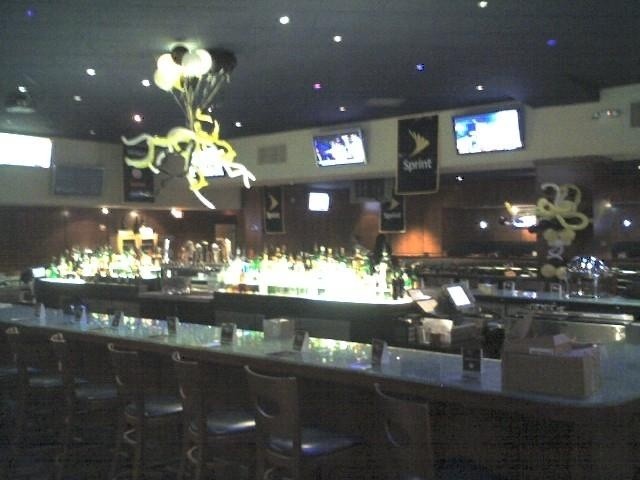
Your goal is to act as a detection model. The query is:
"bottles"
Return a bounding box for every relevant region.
[228,239,421,300]
[50,238,222,282]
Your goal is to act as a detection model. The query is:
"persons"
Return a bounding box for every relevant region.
[374,234,393,267]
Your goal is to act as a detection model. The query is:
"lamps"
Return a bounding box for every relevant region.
[4,82,38,115]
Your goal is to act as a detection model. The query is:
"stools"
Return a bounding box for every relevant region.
[170,351,256,480]
[244,366,367,480]
[0,325,128,479]
[107,342,186,480]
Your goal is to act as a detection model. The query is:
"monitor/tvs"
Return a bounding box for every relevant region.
[306,191,332,211]
[313,126,368,169]
[441,282,476,310]
[452,107,524,156]
[0,131,54,170]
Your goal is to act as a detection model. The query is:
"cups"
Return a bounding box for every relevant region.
[503,280,515,295]
[551,283,561,297]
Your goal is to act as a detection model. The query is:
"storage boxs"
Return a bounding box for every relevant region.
[491,335,604,401]
